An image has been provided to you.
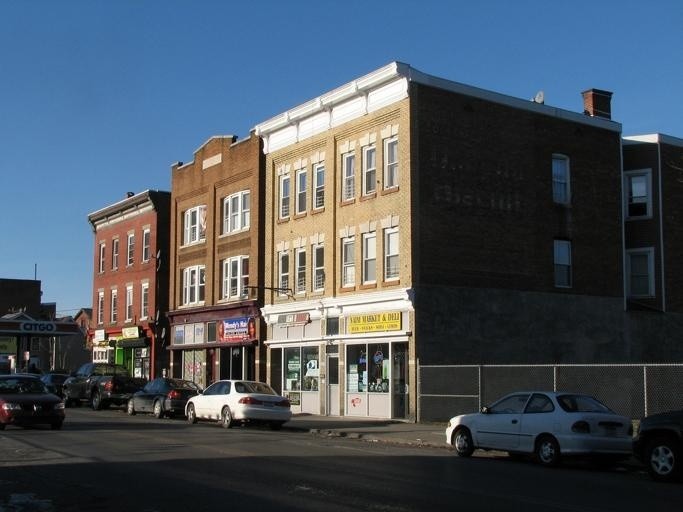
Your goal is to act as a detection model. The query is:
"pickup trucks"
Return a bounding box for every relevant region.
[64,362,145,410]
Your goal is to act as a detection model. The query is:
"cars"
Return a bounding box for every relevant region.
[127,378,203,417]
[12,375,66,429]
[184,378,291,430]
[633,410,683,477]
[42,374,72,397]
[445,392,633,467]
